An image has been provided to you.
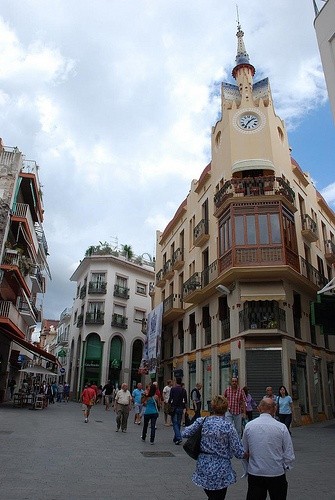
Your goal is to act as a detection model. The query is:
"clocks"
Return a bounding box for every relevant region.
[232,107,266,135]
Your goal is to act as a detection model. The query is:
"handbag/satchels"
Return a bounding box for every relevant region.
[275,397,281,420]
[163,402,175,415]
[189,399,201,410]
[183,418,205,460]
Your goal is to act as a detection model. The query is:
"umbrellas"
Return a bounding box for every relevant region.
[18,364,58,393]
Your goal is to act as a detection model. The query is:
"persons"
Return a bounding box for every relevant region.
[182,394,249,500]
[162,384,174,426]
[224,377,247,441]
[276,385,295,437]
[81,383,97,424]
[169,377,185,444]
[264,386,277,419]
[142,384,152,405]
[8,378,71,403]
[241,386,260,422]
[101,380,113,411]
[141,385,159,444]
[98,385,103,402]
[132,383,144,425]
[190,382,201,425]
[91,382,98,404]
[241,397,296,500]
[152,382,162,430]
[115,383,132,432]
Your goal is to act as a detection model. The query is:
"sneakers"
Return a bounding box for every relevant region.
[149,440,154,445]
[105,407,176,433]
[173,438,182,445]
[140,435,145,442]
[84,417,89,423]
